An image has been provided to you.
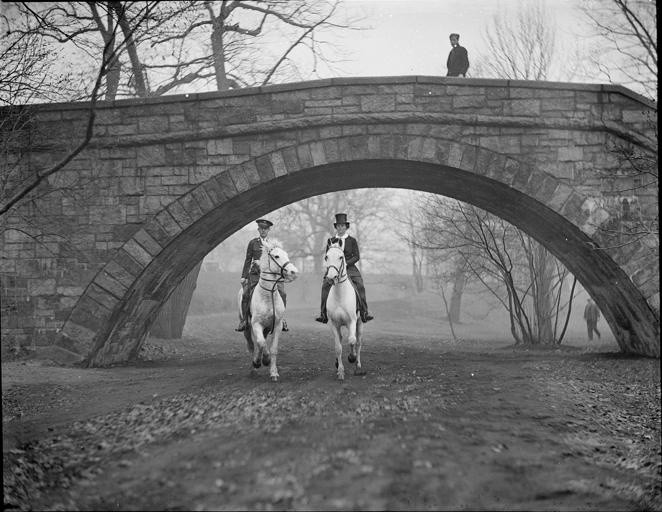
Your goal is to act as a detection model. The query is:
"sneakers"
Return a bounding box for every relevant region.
[316,317,328,323]
[362,316,373,321]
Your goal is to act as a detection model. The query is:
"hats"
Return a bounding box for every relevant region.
[256,219,273,228]
[334,214,349,229]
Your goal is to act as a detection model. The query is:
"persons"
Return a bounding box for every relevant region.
[583,297,602,341]
[314,213,374,324]
[235,219,290,332]
[445,34,470,78]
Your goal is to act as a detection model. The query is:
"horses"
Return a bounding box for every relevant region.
[237,237,301,382]
[323,239,363,380]
